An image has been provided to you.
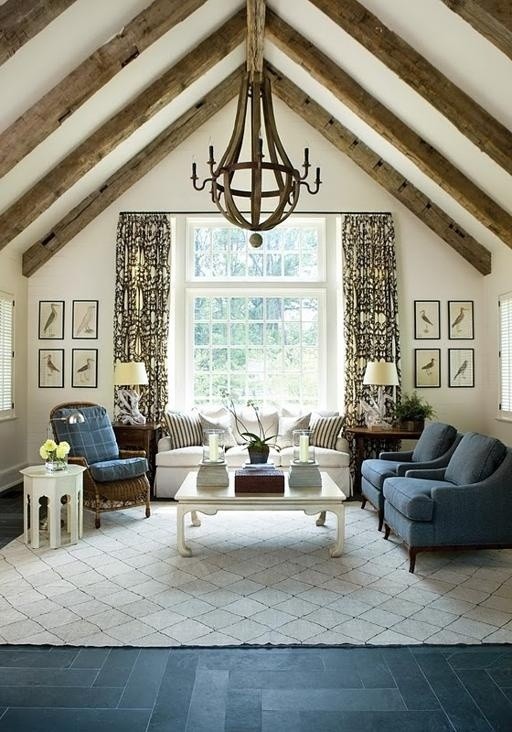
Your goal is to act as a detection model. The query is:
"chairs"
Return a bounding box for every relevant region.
[52,402,150,528]
[359,421,512,572]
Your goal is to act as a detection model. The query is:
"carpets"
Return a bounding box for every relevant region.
[1,500,511,647]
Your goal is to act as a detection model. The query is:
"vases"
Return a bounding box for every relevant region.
[46,459,69,472]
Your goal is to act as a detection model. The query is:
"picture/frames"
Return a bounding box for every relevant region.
[414,300,475,389]
[37,299,99,387]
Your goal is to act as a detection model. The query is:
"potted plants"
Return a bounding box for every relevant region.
[223,397,282,465]
[395,392,438,432]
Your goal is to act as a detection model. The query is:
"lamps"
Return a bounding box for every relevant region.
[114,363,149,424]
[191,0,322,249]
[363,362,400,429]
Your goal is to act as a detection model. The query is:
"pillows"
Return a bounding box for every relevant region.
[166,400,346,449]
[53,406,120,460]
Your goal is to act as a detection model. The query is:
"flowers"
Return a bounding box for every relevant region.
[41,438,70,460]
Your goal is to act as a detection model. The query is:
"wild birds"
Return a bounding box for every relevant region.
[451,307,470,333]
[420,358,438,377]
[42,353,62,379]
[418,309,435,336]
[76,305,95,338]
[77,358,95,384]
[453,359,469,382]
[43,302,61,338]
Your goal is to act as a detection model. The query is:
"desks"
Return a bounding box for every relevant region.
[345,428,422,508]
[111,422,164,469]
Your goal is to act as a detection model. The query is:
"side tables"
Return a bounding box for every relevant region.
[19,464,88,550]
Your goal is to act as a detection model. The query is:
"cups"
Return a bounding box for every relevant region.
[202,429,225,465]
[293,429,314,465]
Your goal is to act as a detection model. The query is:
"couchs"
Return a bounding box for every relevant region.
[152,436,351,500]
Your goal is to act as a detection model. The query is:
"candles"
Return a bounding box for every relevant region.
[208,435,218,461]
[299,433,309,464]
